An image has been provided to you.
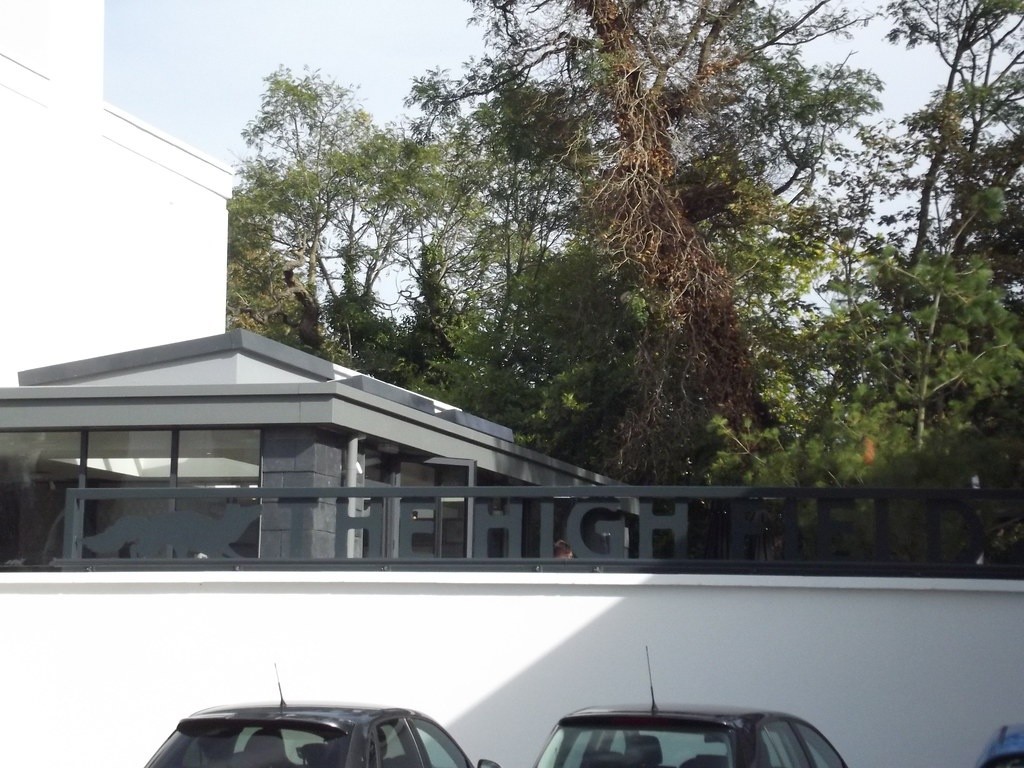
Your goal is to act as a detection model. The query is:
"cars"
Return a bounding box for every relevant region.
[532,706,849,768]
[144,704,503,768]
[974,723,1024,768]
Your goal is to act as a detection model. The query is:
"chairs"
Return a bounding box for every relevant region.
[581,750,624,768]
[679,754,729,768]
[361,726,408,768]
[625,735,662,768]
[228,727,295,768]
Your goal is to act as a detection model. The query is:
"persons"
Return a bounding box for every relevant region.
[553,540,573,558]
